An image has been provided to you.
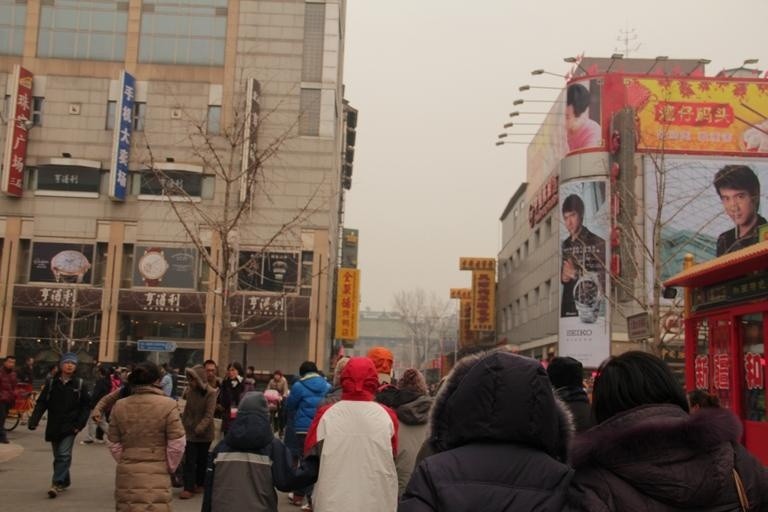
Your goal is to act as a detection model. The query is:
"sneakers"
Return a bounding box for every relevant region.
[287,493,303,505]
[301,504,311,512]
[47,485,57,497]
[193,485,204,493]
[179,491,192,499]
[0,435,9,444]
[58,484,70,491]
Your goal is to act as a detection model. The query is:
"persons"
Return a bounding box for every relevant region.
[199,388,324,512]
[28,351,93,499]
[1,345,726,511]
[107,360,188,511]
[559,192,606,317]
[568,351,765,512]
[244,252,260,291]
[393,349,609,511]
[565,82,603,151]
[295,356,399,512]
[711,165,766,258]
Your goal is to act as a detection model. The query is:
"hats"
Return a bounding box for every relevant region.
[59,352,77,368]
[333,358,350,386]
[397,368,427,396]
[237,392,270,420]
[299,361,317,376]
[546,356,582,388]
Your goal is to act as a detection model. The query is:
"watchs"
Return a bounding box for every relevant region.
[49,249,94,285]
[137,246,170,288]
[572,272,600,324]
